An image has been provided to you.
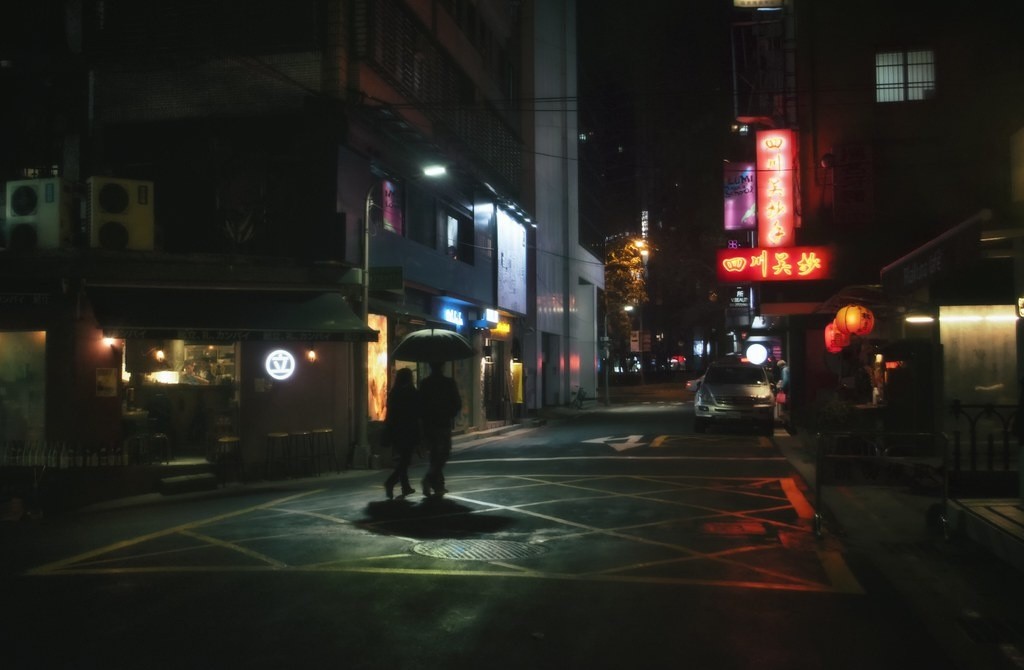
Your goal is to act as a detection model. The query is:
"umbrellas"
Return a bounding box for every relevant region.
[392,329,479,362]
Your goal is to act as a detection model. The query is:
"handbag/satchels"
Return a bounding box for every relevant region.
[776,390,786,403]
[379,427,392,448]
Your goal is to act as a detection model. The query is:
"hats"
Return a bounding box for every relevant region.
[776,360,786,366]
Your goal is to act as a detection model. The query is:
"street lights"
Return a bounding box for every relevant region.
[604,305,635,404]
[350,162,449,467]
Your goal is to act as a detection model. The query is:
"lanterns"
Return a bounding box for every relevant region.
[824,305,874,353]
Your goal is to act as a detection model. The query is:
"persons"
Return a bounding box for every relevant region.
[379,358,462,499]
[776,360,797,435]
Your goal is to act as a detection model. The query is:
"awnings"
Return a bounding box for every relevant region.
[366,296,456,332]
[0,291,80,332]
[87,283,380,345]
[809,209,1024,317]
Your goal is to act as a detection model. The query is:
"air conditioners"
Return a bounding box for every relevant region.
[80,175,155,252]
[4,177,72,250]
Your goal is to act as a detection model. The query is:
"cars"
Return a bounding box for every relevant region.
[680,372,705,400]
[693,358,778,437]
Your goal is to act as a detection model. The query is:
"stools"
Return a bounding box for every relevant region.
[215,437,246,487]
[263,426,338,479]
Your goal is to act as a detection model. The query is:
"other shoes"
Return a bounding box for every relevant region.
[384,481,393,499]
[402,487,415,496]
[436,488,448,498]
[421,479,432,498]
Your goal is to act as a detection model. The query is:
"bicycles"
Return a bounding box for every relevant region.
[570,384,588,411]
